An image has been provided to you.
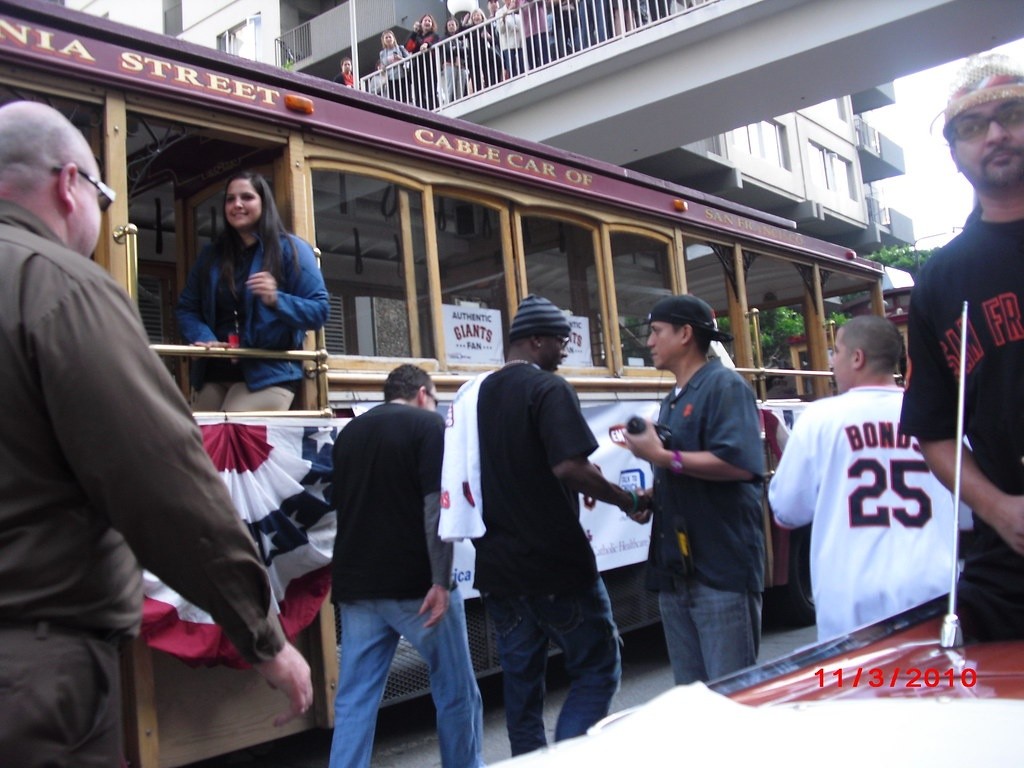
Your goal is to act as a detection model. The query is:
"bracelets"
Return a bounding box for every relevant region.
[670,449,684,474]
[626,490,639,514]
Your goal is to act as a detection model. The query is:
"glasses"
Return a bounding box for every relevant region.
[948,103,1023,143]
[551,333,570,348]
[53,166,117,211]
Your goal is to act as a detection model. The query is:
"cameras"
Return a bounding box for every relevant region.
[627,416,672,451]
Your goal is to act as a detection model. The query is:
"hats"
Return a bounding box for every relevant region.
[648,292,733,341]
[943,54,1024,142]
[509,293,571,343]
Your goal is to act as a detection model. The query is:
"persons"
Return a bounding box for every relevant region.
[174,168,331,413]
[333,56,355,88]
[897,52,1024,646]
[0,101,314,768]
[622,295,767,688]
[768,314,958,644]
[370,0,695,111]
[434,294,653,757]
[328,363,486,768]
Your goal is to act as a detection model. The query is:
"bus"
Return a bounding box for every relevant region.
[0,0,886,768]
